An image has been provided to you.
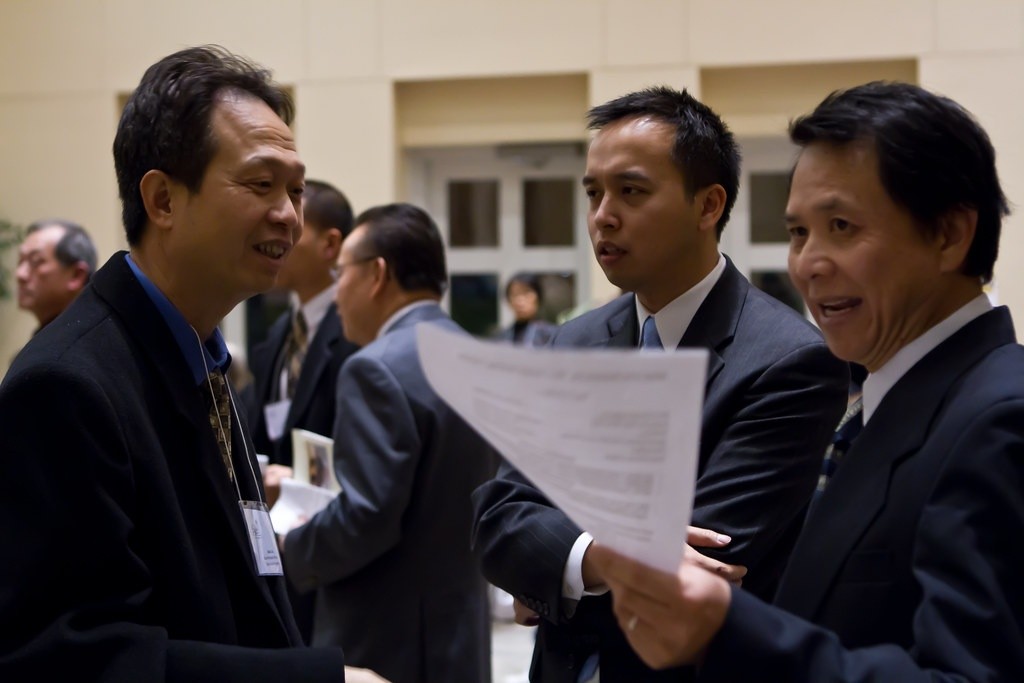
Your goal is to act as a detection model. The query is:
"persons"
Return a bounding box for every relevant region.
[279,202,491,682]
[16,219,98,340]
[498,272,562,344]
[240,179,360,507]
[473,87,849,683]
[0,47,390,683]
[610,77,1024,683]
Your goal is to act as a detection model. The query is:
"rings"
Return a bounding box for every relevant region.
[628,614,638,630]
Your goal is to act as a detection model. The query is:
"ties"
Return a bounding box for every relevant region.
[283,309,307,403]
[641,317,663,349]
[204,367,232,477]
[807,395,863,510]
